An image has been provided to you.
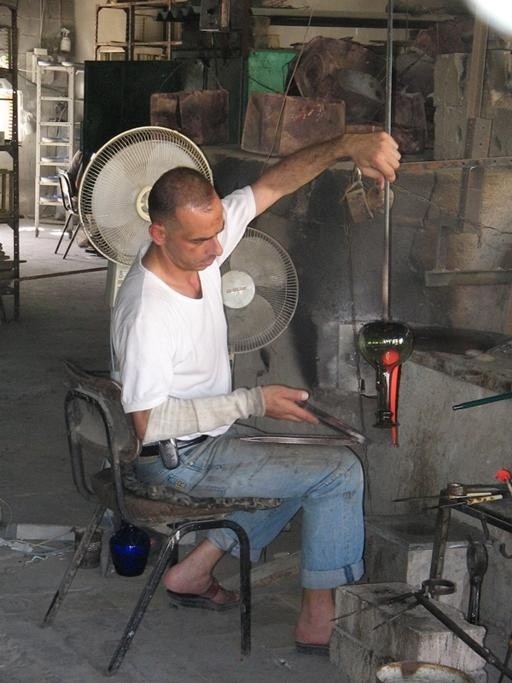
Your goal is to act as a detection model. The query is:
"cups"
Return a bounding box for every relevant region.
[73,529,102,568]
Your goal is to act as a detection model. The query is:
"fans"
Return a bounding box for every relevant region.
[2,124,218,544]
[216,227,299,388]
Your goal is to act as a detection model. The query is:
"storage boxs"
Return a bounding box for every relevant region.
[238,85,347,166]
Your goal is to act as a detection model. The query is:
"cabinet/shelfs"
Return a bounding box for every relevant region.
[198,3,461,181]
[0,0,235,320]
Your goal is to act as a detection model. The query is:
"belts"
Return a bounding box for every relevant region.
[139,435,209,456]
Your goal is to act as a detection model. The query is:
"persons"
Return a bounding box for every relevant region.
[111,127,404,658]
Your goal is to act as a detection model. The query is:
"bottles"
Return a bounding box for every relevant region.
[108,521,149,575]
[357,319,413,428]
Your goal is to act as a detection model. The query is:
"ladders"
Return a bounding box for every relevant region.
[35,65,74,240]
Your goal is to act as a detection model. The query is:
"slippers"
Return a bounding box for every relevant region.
[166,579,240,612]
[294,640,329,656]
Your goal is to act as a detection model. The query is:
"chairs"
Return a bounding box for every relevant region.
[40,385,282,674]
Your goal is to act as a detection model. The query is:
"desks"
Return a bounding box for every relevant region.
[417,480,511,678]
